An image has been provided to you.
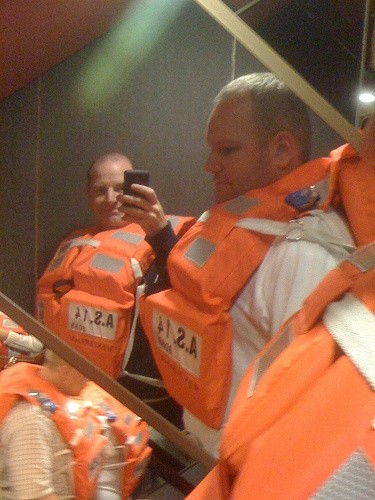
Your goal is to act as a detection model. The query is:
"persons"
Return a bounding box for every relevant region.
[141,69,357,500]
[1,153,197,500]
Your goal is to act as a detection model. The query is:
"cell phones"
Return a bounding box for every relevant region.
[121,170,149,208]
